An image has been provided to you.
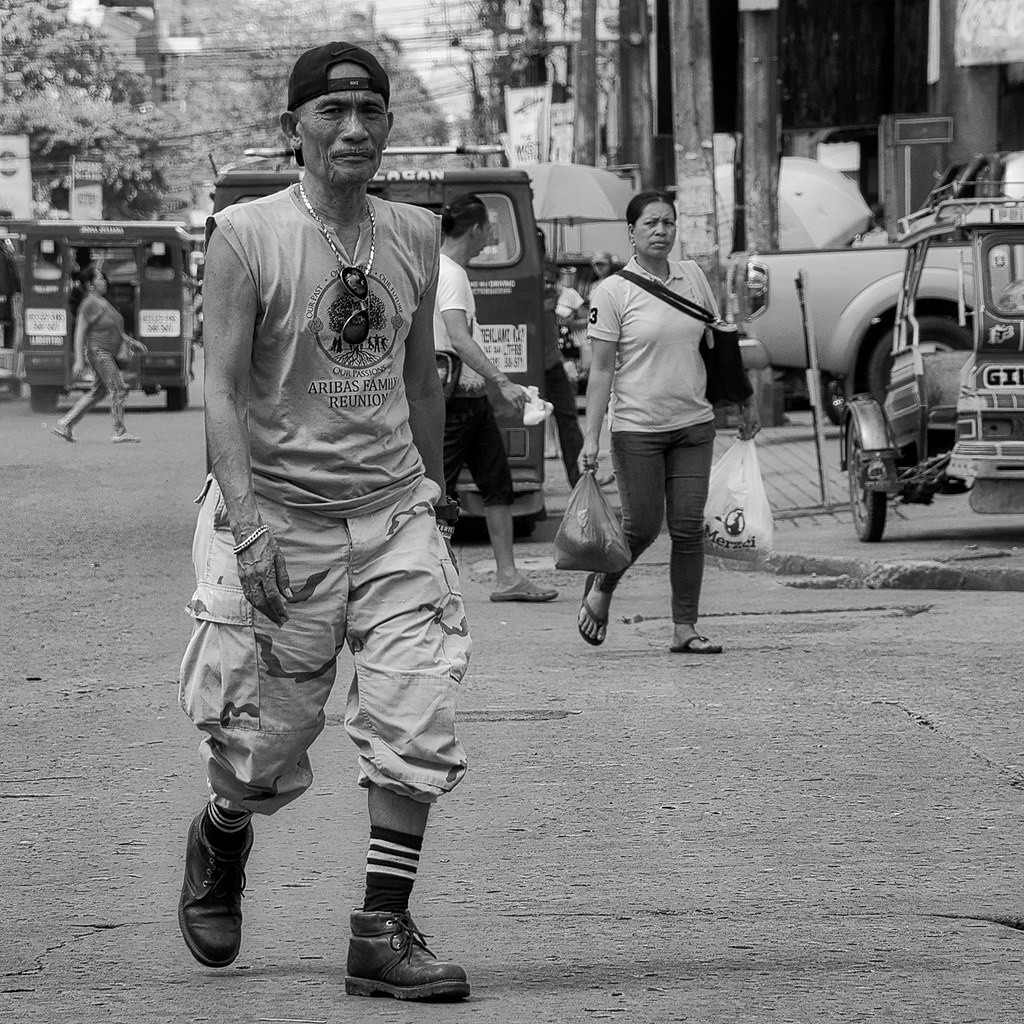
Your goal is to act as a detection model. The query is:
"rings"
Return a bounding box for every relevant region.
[521,401,524,404]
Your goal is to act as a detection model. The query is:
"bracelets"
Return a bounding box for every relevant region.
[438,524,457,539]
[232,524,269,553]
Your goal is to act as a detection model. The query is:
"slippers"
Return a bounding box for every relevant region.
[670,636,722,654]
[578,572,609,646]
[490,576,558,601]
[51,427,77,443]
[112,432,140,444]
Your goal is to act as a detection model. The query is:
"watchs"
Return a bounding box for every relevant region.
[432,499,460,522]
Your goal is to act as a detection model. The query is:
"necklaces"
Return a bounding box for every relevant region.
[298,182,377,277]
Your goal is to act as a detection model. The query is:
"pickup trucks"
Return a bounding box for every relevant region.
[742,152,1024,494]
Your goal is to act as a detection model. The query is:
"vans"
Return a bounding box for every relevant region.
[9,221,195,412]
[203,146,557,539]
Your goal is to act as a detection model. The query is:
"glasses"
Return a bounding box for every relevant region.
[340,267,369,345]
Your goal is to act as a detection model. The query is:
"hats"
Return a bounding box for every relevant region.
[287,42,390,166]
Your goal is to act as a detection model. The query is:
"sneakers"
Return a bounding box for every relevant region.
[177,806,254,968]
[345,909,471,999]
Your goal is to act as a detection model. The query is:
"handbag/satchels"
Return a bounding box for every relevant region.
[435,349,463,403]
[115,343,135,369]
[700,321,754,407]
[551,471,632,572]
[702,437,775,564]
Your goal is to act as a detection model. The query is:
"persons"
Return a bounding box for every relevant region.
[53,267,149,445]
[430,193,560,602]
[179,43,472,1003]
[575,193,723,653]
[533,225,615,490]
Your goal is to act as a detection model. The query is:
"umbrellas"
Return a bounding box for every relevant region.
[503,161,636,260]
[674,156,875,250]
